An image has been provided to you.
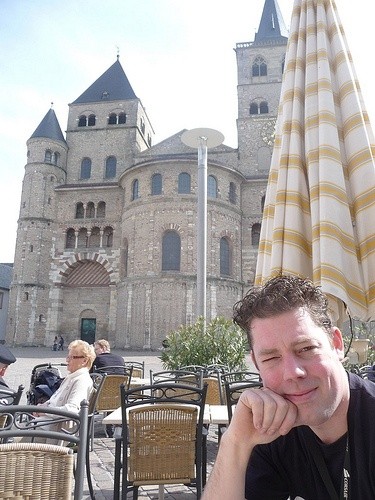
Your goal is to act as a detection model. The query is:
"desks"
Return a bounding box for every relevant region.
[210,404,238,451]
[102,404,210,500]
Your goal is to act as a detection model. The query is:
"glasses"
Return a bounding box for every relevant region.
[65,355,85,359]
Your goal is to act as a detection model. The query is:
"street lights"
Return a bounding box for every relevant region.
[180,126,224,346]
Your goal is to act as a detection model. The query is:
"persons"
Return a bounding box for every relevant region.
[0,343,16,380]
[32,340,96,419]
[198,273,375,500]
[52,336,58,351]
[58,337,65,351]
[89,339,125,380]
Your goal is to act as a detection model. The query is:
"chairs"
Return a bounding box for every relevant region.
[0,361,264,500]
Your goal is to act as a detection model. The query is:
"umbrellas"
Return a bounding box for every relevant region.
[255,0,375,336]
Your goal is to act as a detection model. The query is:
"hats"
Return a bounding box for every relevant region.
[0,344,16,365]
